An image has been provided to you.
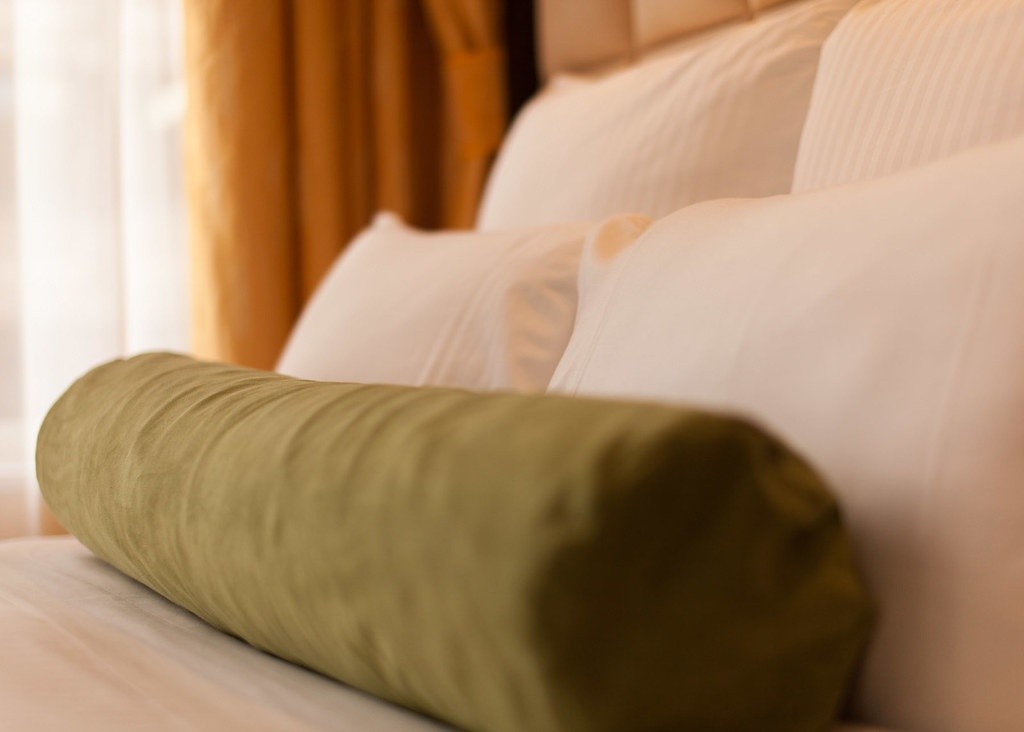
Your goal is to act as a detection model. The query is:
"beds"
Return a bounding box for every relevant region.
[0,0,1024,732]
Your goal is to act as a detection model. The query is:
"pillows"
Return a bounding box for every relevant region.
[792,0,1024,190]
[543,150,1024,732]
[275,213,580,393]
[474,0,851,229]
[32,352,879,732]
